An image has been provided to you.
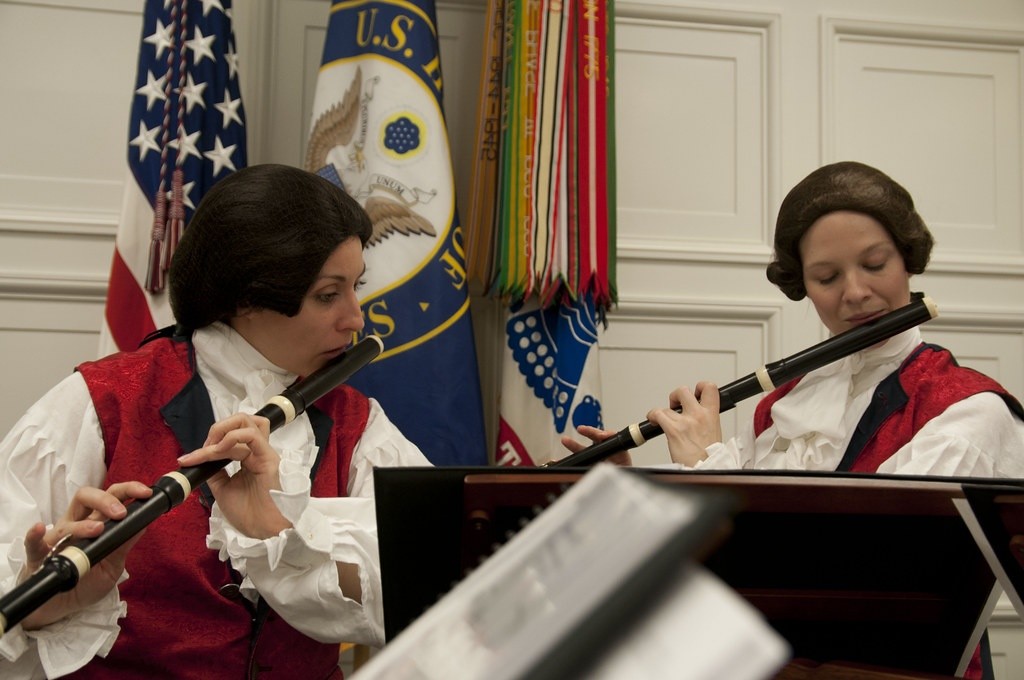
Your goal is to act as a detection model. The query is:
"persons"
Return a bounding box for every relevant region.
[0,164,437,680]
[549,161,1024,680]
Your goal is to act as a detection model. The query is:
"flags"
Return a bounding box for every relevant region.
[98,0,250,363]
[303,0,488,467]
[464,0,618,469]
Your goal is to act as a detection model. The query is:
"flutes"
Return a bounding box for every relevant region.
[535,295,940,467]
[0,332,385,639]
